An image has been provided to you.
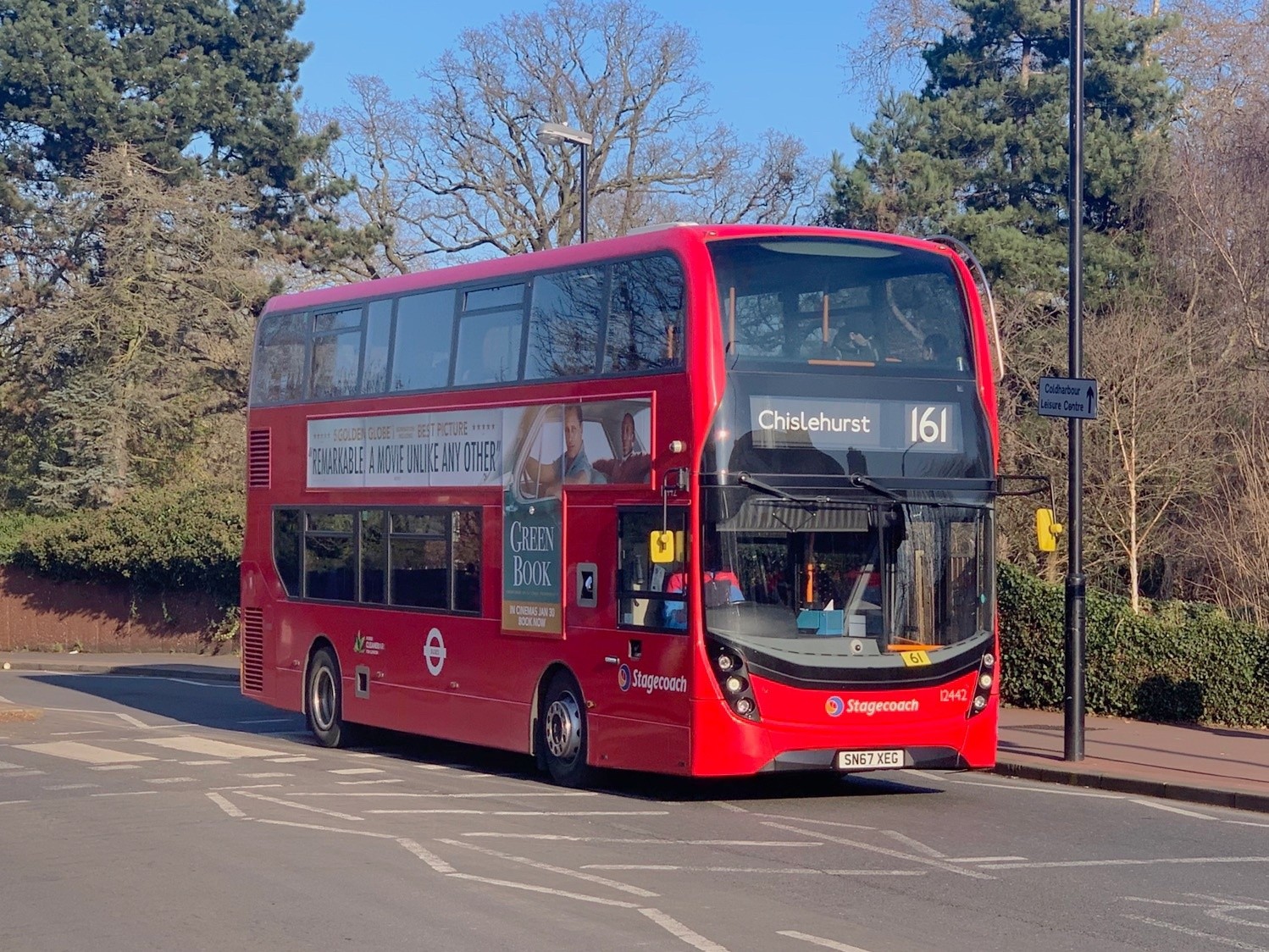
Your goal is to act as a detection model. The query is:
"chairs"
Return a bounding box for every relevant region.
[287,566,481,611]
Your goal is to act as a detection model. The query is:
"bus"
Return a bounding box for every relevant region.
[239,219,1064,790]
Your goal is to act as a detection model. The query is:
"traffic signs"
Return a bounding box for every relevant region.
[1037,375,1097,420]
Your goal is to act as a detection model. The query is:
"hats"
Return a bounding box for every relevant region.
[845,313,876,336]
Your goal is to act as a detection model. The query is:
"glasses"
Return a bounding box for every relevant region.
[854,331,870,340]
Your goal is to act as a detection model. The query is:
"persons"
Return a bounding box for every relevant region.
[527,404,654,503]
[827,315,890,362]
[921,333,952,364]
[660,536,746,628]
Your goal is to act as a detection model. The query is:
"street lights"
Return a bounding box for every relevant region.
[535,122,594,243]
